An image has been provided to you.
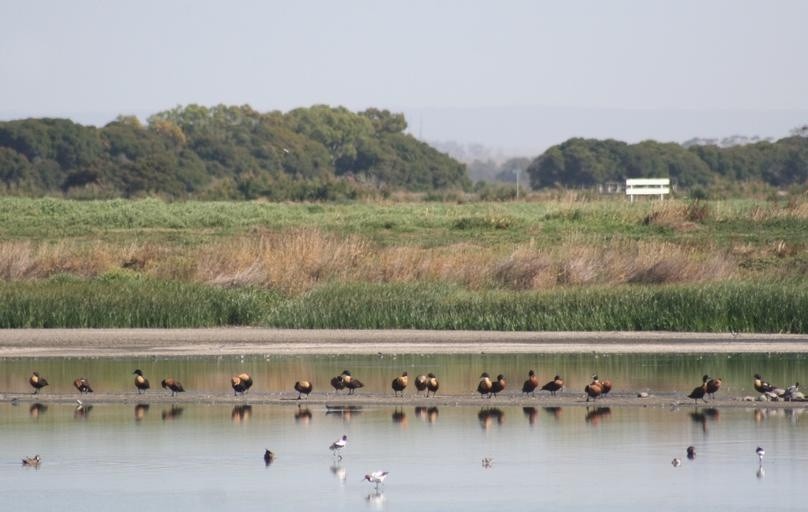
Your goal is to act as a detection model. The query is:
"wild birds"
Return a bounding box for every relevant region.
[74,377,93,396]
[752,372,800,402]
[132,369,184,397]
[360,470,390,493]
[329,435,348,460]
[332,368,362,395]
[295,380,312,398]
[687,374,722,405]
[30,370,49,394]
[477,368,612,402]
[754,445,767,464]
[392,370,442,398]
[229,371,254,397]
